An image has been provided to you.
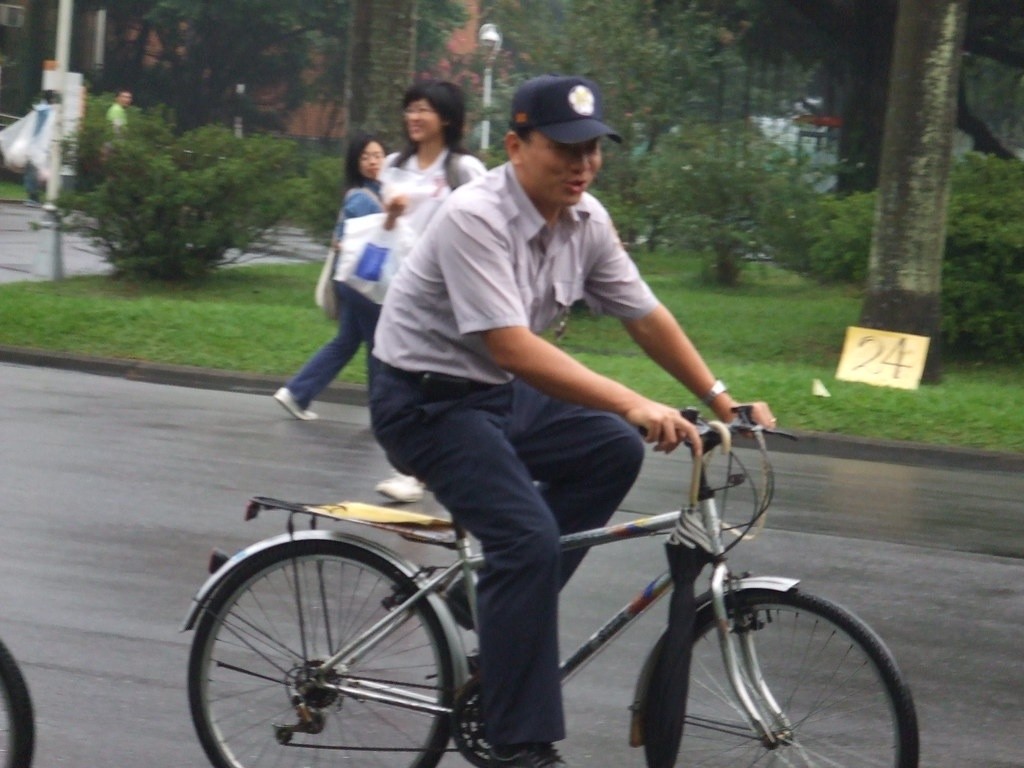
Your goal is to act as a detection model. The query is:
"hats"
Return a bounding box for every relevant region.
[507,74,625,149]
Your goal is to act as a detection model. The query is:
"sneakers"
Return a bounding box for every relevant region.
[274,387,320,422]
[372,470,427,503]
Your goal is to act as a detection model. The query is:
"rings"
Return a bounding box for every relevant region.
[770,418,777,423]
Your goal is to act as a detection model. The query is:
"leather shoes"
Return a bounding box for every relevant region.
[491,741,571,768]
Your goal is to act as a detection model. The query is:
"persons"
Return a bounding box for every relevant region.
[369,74,775,768]
[273,81,489,505]
[106,90,133,138]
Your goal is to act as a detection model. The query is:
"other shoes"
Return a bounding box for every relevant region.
[22,197,43,210]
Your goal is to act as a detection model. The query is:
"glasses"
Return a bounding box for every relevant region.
[358,155,386,162]
[403,106,437,116]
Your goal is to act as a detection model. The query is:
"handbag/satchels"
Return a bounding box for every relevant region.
[315,248,342,322]
[332,213,419,307]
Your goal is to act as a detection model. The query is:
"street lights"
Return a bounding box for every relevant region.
[478,22,503,152]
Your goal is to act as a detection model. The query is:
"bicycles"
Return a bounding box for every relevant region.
[180,402,921,768]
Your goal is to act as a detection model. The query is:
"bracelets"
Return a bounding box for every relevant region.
[694,380,729,406]
